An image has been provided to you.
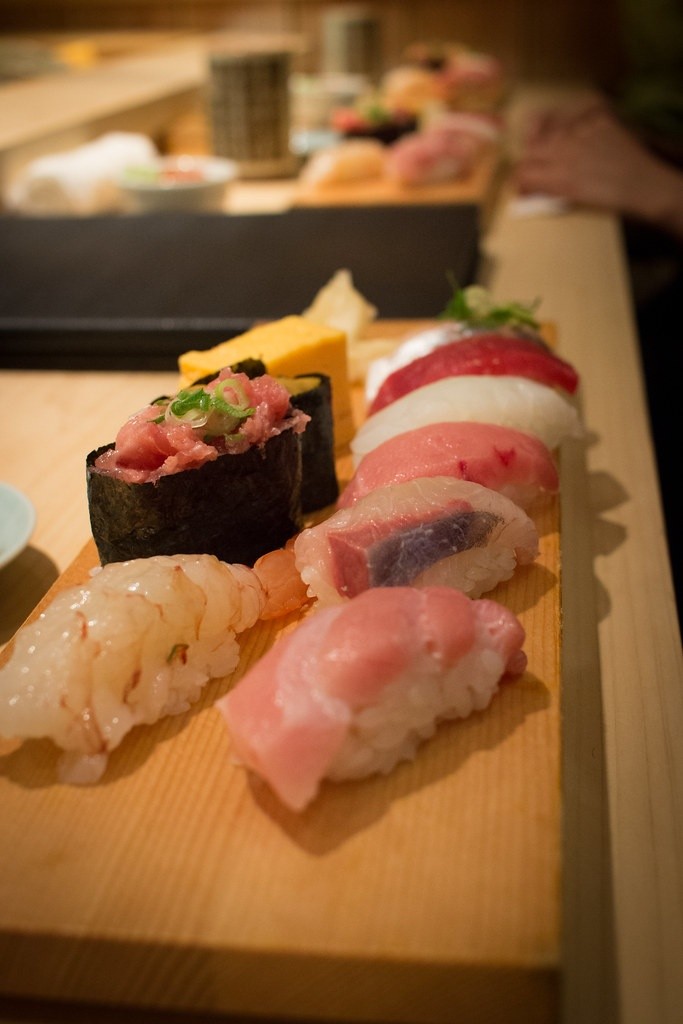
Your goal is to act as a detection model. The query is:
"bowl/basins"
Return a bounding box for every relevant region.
[116,156,239,214]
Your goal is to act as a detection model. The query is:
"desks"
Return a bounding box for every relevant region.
[0,81,683,1024]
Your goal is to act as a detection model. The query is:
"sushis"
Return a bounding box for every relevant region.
[299,53,507,191]
[0,272,578,813]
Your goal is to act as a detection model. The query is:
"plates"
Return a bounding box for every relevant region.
[205,132,342,179]
[0,485,35,569]
